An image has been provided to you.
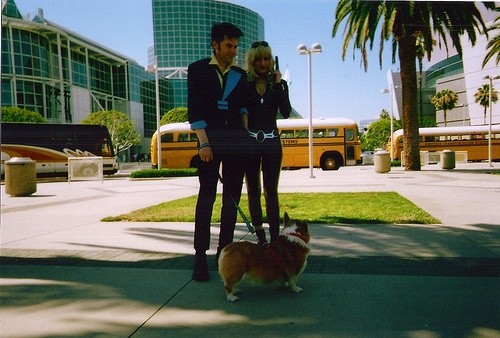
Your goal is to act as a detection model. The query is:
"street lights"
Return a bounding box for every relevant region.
[144,55,161,170]
[381,84,399,162]
[297,43,322,180]
[482,75,500,167]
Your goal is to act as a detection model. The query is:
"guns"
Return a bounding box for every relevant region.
[272,55,279,81]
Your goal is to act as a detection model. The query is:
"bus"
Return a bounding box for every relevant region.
[389,125,500,162]
[148,117,360,173]
[1,122,120,176]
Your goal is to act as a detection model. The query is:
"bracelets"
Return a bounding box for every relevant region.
[199,143,209,150]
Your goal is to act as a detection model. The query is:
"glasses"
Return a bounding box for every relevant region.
[251,41,268,48]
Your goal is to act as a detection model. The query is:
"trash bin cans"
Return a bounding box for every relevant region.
[374,150,391,173]
[441,149,456,169]
[4,158,37,196]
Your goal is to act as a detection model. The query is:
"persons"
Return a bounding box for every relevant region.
[245,41,291,245]
[186,22,250,282]
[130,153,151,162]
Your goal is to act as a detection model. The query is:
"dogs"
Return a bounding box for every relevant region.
[218,211,311,303]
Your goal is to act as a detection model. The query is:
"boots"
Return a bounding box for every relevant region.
[255,229,267,243]
[269,227,279,243]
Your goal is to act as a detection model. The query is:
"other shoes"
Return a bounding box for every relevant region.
[193,253,209,281]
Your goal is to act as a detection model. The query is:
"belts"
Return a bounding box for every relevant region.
[248,129,279,142]
